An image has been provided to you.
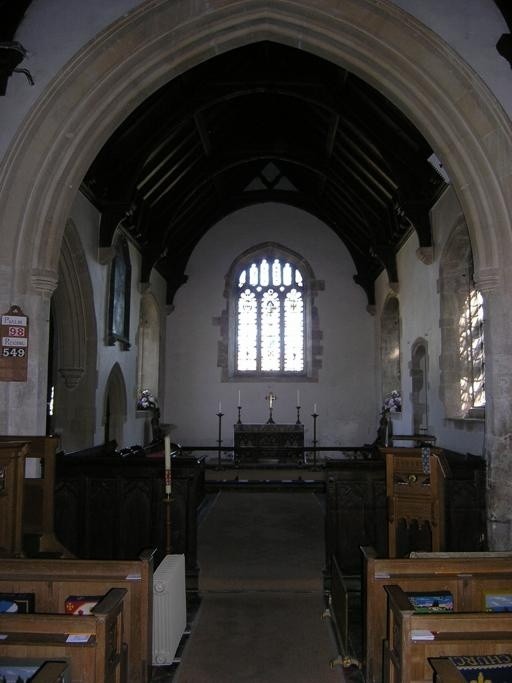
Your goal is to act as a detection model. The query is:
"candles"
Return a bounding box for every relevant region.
[269,394,272,408]
[238,389,240,406]
[296,388,300,406]
[218,399,221,413]
[165,436,172,500]
[313,403,316,412]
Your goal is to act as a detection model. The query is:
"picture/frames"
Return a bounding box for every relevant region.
[107,232,132,351]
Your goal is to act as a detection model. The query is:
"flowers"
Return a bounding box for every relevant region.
[384,390,401,412]
[137,390,152,408]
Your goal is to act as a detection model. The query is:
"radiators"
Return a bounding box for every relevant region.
[153,554,190,667]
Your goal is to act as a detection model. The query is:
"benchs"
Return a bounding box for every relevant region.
[383,583,512,683]
[0,548,158,682]
[3,661,68,682]
[0,587,129,682]
[360,545,512,683]
[429,654,511,682]
